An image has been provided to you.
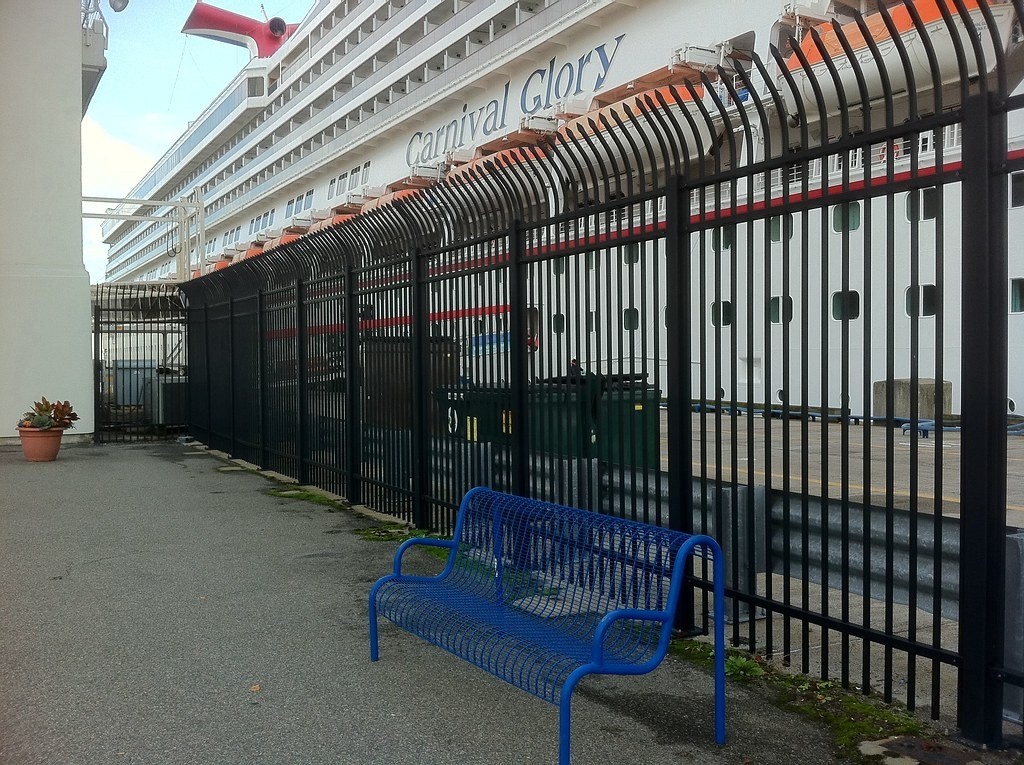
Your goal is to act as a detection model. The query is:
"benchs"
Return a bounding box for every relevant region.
[367,487,725,765]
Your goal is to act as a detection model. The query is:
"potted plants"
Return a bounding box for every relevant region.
[14,393,80,463]
